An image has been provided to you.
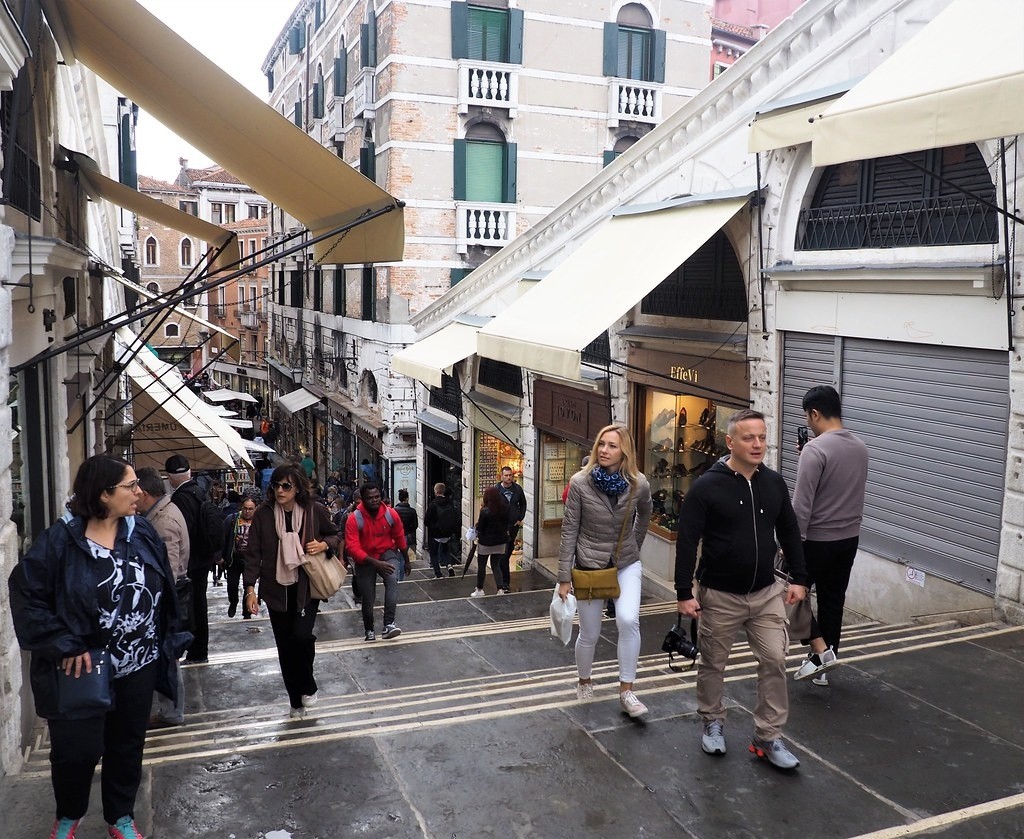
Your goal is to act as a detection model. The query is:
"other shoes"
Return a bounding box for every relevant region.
[148,715,178,729]
[228,603,237,618]
[243,613,251,619]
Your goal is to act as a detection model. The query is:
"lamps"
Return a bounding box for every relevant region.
[288,339,358,385]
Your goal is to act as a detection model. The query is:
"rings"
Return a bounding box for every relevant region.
[311,549,314,553]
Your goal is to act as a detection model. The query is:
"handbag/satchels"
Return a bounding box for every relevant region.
[57,646,111,721]
[781,590,811,640]
[301,552,348,599]
[571,567,621,606]
[550,583,577,647]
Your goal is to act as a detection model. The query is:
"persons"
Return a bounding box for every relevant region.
[8,453,183,839]
[133,466,190,731]
[675,408,807,772]
[344,483,411,641]
[424,482,455,579]
[184,370,265,423]
[166,455,218,664]
[471,486,508,597]
[243,463,340,719]
[212,418,391,588]
[495,466,527,593]
[783,385,870,686]
[558,423,650,717]
[395,489,418,576]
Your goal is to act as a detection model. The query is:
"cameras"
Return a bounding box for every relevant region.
[662,625,700,659]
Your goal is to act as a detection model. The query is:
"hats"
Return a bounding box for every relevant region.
[165,455,189,473]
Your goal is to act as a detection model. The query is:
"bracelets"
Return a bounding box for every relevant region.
[245,592,256,599]
[322,542,330,551]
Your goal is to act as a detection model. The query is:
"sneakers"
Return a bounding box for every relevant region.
[301,691,318,707]
[702,720,802,770]
[793,645,837,686]
[290,707,307,718]
[354,600,402,643]
[108,815,144,839]
[432,563,511,596]
[577,682,650,717]
[49,818,80,839]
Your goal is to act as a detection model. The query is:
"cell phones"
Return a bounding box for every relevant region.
[798,426,808,452]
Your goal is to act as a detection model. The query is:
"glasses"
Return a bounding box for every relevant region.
[241,506,254,512]
[106,478,141,492]
[271,481,296,492]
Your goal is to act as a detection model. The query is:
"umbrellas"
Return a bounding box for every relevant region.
[461,538,477,579]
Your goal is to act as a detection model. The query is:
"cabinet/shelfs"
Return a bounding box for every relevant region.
[542,457,579,528]
[648,390,729,528]
[473,430,523,555]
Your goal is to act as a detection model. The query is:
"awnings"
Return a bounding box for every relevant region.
[38,0,407,266]
[60,146,243,272]
[54,265,405,471]
[389,0,1024,388]
[278,388,323,419]
